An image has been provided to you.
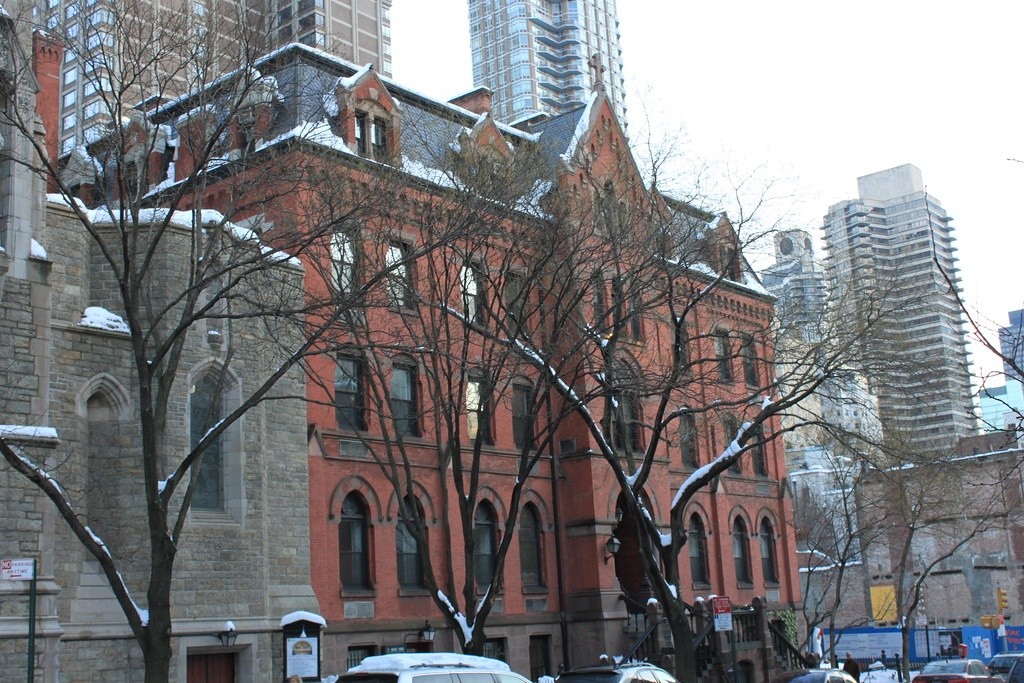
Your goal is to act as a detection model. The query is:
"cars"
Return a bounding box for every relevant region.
[911,658,1002,683]
[771,668,857,683]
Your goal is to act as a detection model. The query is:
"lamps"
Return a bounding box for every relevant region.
[418,620,436,642]
[213,620,237,648]
[603,533,622,564]
[615,505,624,528]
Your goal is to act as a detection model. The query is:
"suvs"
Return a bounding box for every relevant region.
[986,651,1024,683]
[335,656,535,683]
[554,662,681,683]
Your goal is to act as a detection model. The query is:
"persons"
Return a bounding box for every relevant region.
[842,651,861,683]
